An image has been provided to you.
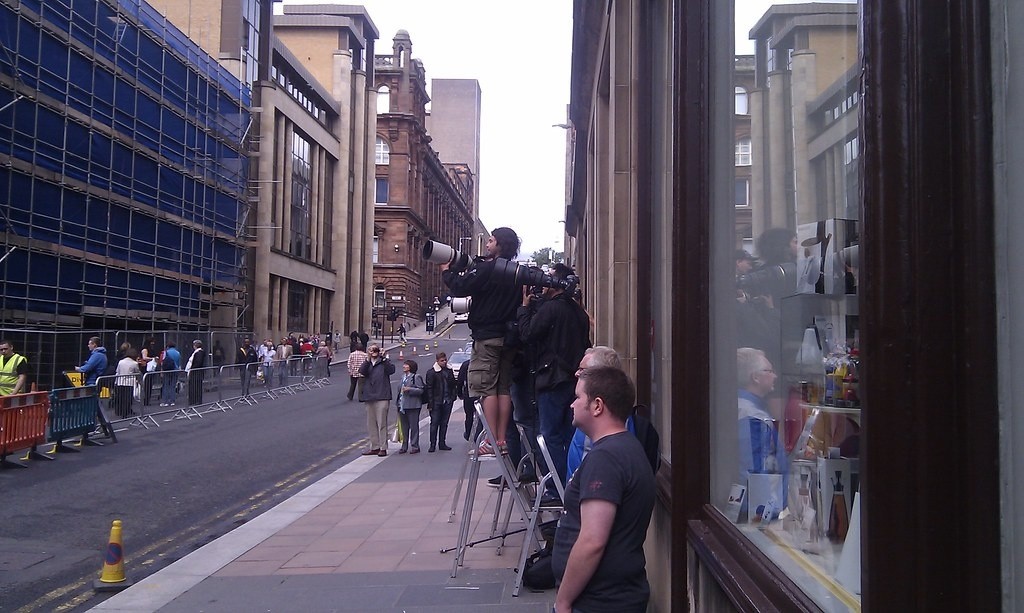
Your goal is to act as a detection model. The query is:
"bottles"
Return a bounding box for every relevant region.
[795,327,862,408]
[794,474,819,548]
[828,471,849,544]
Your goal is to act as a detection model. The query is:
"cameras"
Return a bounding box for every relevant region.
[450,296,473,313]
[422,241,485,273]
[372,347,380,352]
[492,258,579,300]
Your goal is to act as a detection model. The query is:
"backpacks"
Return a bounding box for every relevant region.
[631,403,660,477]
[161,349,176,371]
[514,544,557,594]
[412,372,429,405]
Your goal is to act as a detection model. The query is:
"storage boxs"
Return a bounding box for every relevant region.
[795,218,858,293]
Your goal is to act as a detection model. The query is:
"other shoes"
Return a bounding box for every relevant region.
[439,444,452,450]
[159,403,169,407]
[378,449,387,456]
[530,493,564,507]
[428,444,436,452]
[361,447,380,455]
[486,474,540,487]
[170,403,175,407]
[398,448,407,454]
[410,447,420,454]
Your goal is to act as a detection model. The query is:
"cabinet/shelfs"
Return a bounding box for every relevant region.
[776,291,860,555]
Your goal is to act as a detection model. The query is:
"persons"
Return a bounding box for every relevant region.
[397,324,408,343]
[185,339,206,405]
[239,338,258,401]
[566,345,636,483]
[736,227,798,441]
[108,338,181,419]
[0,338,30,461]
[736,347,789,508]
[397,351,484,453]
[550,367,657,613]
[250,331,333,387]
[358,345,396,457]
[350,330,369,347]
[74,336,108,420]
[348,354,369,402]
[210,339,225,377]
[440,227,594,503]
[333,330,342,354]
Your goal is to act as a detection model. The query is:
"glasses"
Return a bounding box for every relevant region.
[761,369,775,374]
[577,367,588,372]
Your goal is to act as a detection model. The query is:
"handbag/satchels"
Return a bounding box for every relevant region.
[146,358,157,372]
[256,366,265,382]
[390,412,404,443]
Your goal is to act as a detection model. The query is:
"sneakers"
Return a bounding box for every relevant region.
[466,437,509,457]
